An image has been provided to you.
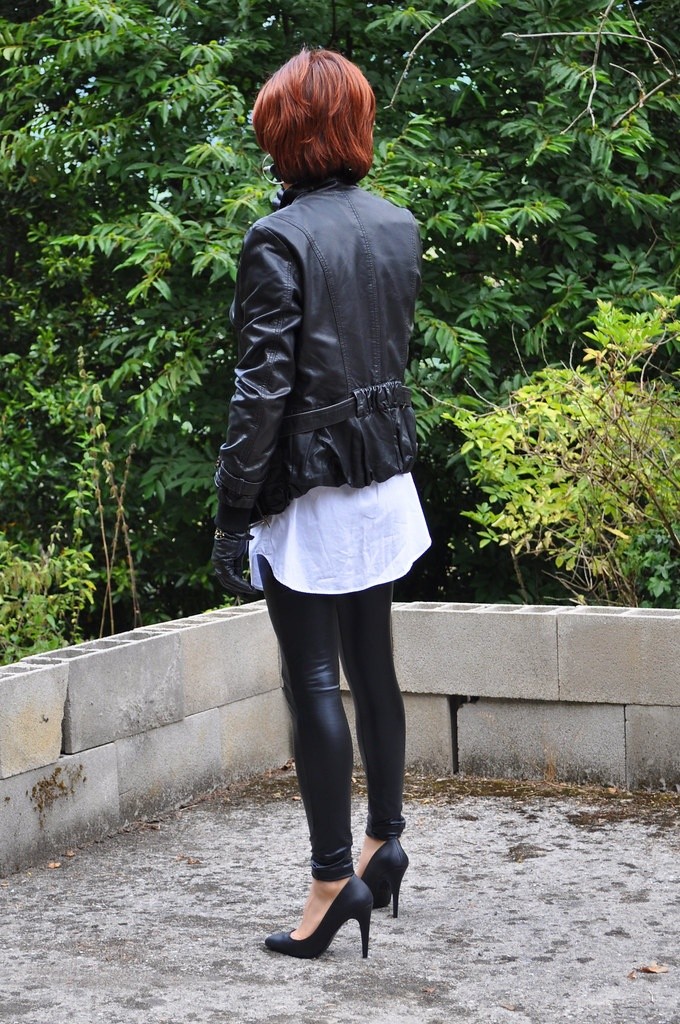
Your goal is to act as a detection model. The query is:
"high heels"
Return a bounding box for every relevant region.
[361,839,409,918]
[265,873,373,959]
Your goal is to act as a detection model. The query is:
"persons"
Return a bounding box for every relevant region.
[210,47,433,959]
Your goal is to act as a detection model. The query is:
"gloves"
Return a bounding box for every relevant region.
[211,526,258,594]
[272,189,284,209]
